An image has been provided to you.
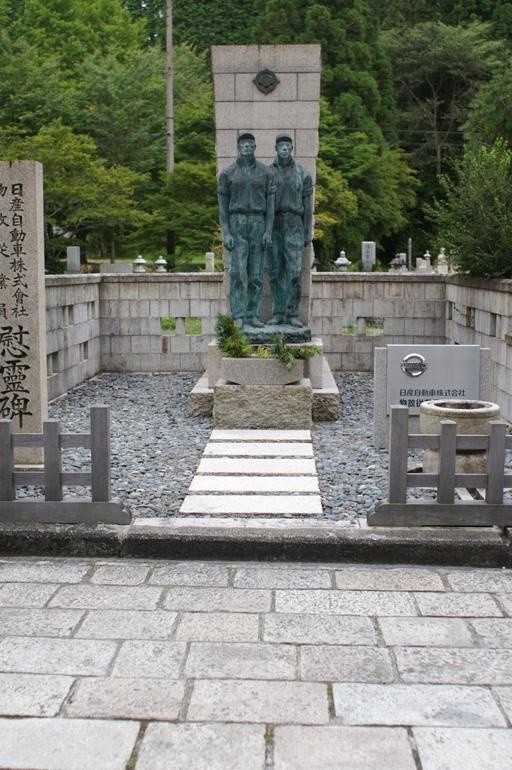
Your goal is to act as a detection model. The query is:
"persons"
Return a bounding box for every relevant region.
[263,132,314,328]
[217,133,278,330]
